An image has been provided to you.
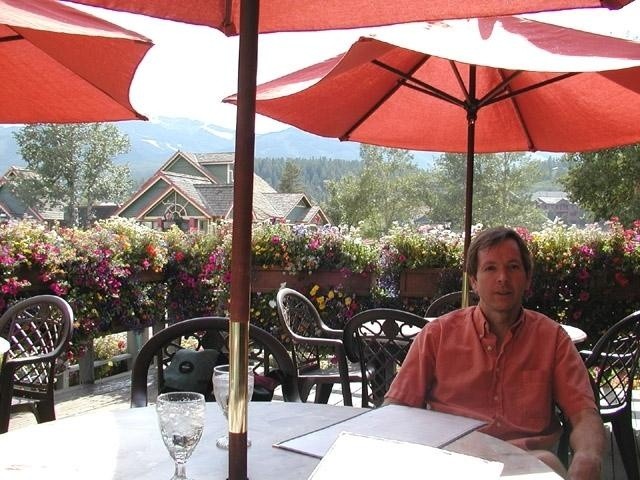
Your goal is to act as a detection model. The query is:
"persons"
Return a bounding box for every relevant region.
[379,224,606,480]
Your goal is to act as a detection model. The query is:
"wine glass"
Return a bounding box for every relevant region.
[212,364,254,450]
[156,392,206,480]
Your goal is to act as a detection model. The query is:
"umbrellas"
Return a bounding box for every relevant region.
[1,0,156,126]
[220,15,640,310]
[65,0,635,479]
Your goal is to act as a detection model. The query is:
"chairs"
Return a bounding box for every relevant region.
[0,288,640,480]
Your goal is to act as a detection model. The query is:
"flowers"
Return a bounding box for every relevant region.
[0,213,640,358]
